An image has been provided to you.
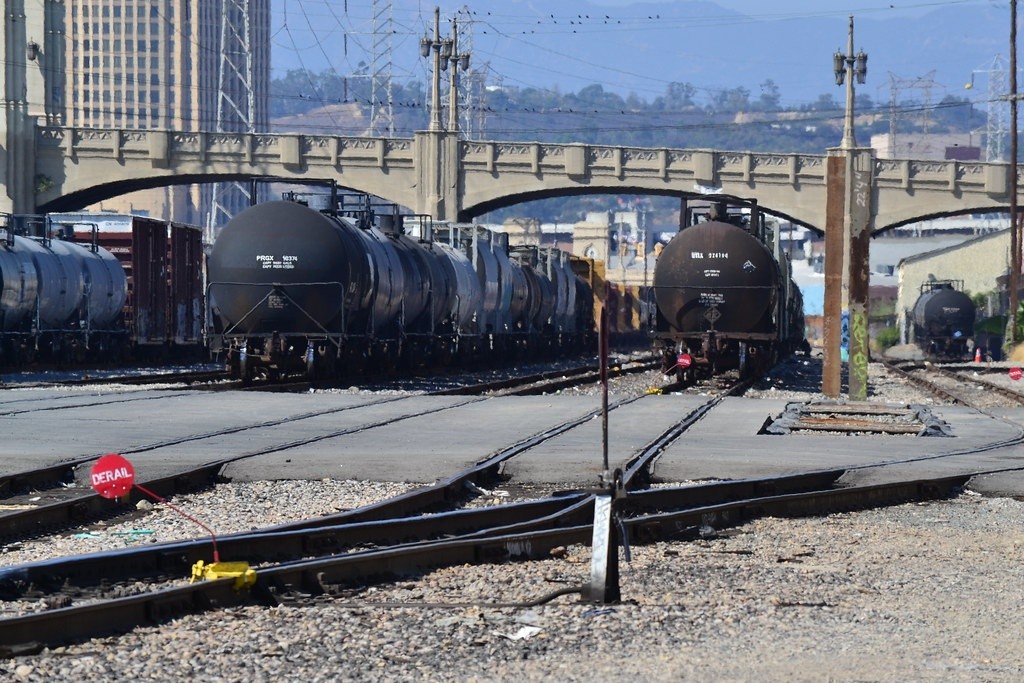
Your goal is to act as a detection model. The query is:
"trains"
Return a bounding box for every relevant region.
[0,208,216,369]
[909,279,975,358]
[647,194,805,380]
[205,173,655,383]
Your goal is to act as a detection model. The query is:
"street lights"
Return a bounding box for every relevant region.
[834,15,867,313]
[440,14,470,221]
[420,6,453,240]
